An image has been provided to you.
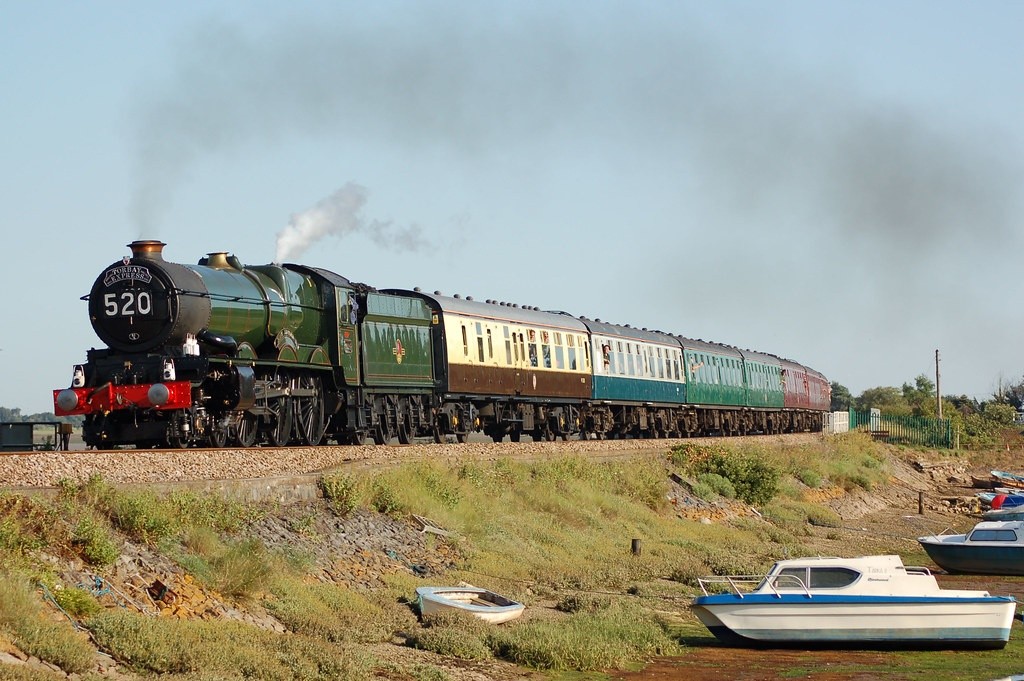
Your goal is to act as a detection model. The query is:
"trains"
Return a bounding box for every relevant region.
[52,241,830,452]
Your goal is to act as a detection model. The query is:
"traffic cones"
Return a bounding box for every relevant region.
[1005,443,1010,450]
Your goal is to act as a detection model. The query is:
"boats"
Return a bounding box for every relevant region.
[688,554,1017,646]
[916,521,1024,574]
[970,470,1024,520]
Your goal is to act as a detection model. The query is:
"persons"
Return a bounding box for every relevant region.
[781,369,786,376]
[530,330,551,368]
[603,345,611,364]
